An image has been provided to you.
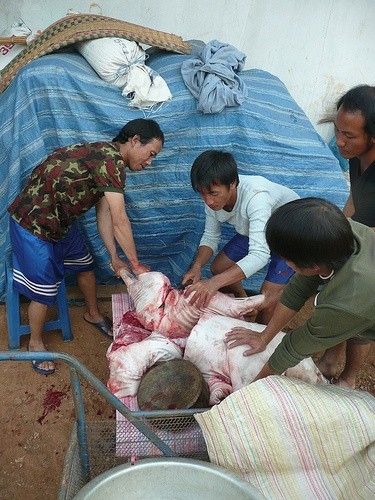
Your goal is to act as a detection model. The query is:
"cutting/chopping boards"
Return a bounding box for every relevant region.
[137,359,210,432]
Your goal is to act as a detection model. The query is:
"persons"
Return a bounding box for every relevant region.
[181,150,302,325]
[7,119,165,374]
[224,196,375,384]
[314,85,375,390]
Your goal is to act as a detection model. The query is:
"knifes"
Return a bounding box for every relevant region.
[158,278,193,308]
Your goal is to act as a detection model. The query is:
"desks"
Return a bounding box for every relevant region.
[111,292,210,458]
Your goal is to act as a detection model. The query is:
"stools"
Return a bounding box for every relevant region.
[4,267,73,351]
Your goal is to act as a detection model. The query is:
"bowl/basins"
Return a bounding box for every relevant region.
[70,457,268,500]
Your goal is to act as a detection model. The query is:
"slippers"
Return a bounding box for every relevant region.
[83,315,113,339]
[32,351,55,374]
